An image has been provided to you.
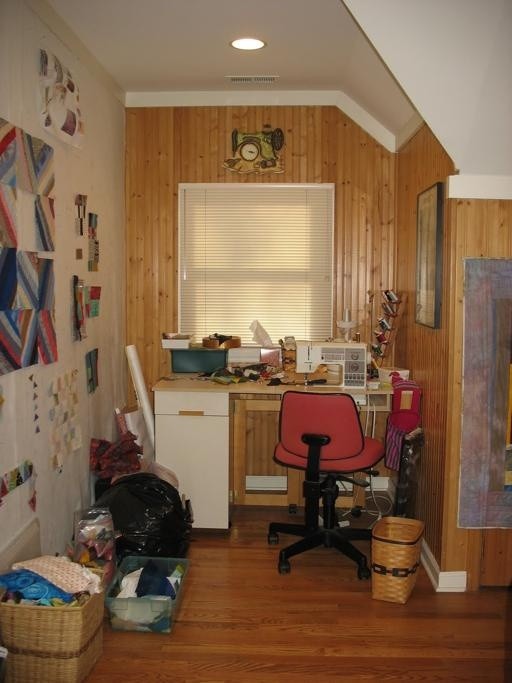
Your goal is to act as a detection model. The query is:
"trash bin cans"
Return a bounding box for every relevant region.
[371,517,425,604]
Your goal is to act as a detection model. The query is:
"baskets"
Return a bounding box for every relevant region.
[3,564,107,682]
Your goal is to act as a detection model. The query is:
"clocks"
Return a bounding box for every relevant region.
[239,140,258,162]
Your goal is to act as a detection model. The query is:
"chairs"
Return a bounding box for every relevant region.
[267,388,388,580]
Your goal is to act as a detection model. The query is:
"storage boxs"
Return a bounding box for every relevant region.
[105,551,192,637]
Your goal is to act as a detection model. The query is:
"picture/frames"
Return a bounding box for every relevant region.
[412,181,445,329]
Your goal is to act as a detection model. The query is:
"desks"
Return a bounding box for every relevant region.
[150,374,396,539]
